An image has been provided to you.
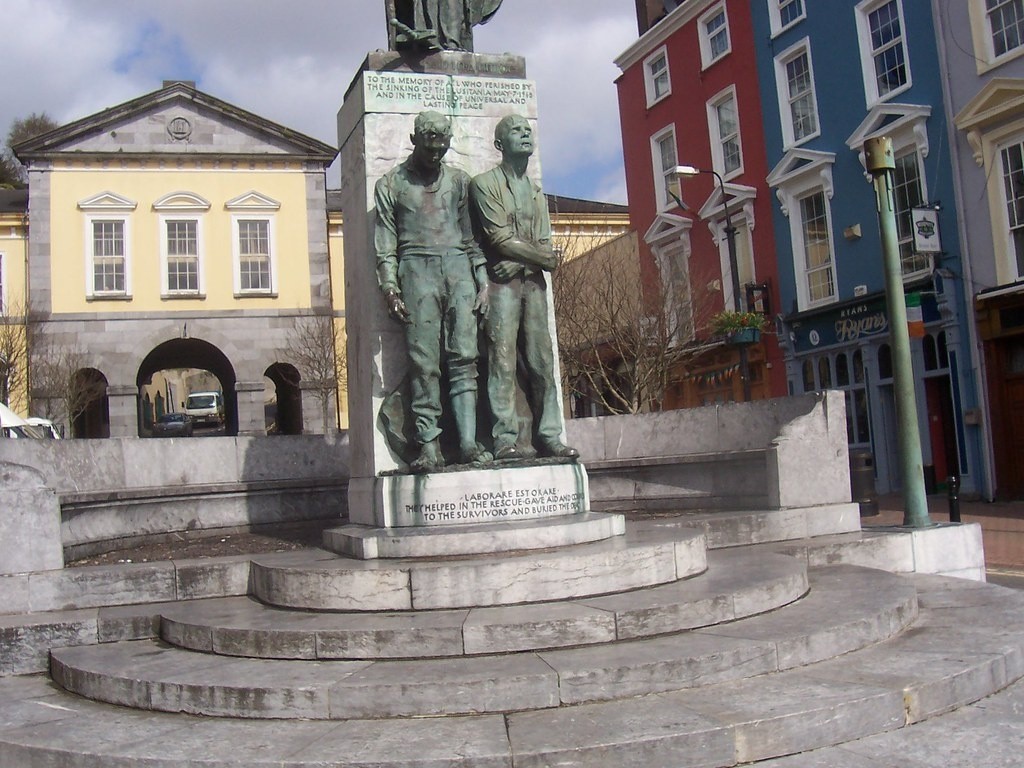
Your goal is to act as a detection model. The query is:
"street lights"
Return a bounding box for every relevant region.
[662,165,751,404]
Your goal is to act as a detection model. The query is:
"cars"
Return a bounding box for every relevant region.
[152,412,194,437]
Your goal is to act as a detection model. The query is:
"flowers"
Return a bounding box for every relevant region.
[707,308,771,336]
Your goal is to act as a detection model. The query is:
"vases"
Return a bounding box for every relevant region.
[725,326,761,346]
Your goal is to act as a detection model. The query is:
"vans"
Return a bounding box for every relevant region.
[0,401,40,438]
[181,392,223,425]
[10,418,62,439]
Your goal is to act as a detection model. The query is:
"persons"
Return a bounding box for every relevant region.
[470,114,580,459]
[374,112,493,467]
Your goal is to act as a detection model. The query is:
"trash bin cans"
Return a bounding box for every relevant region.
[849,449,879,517]
